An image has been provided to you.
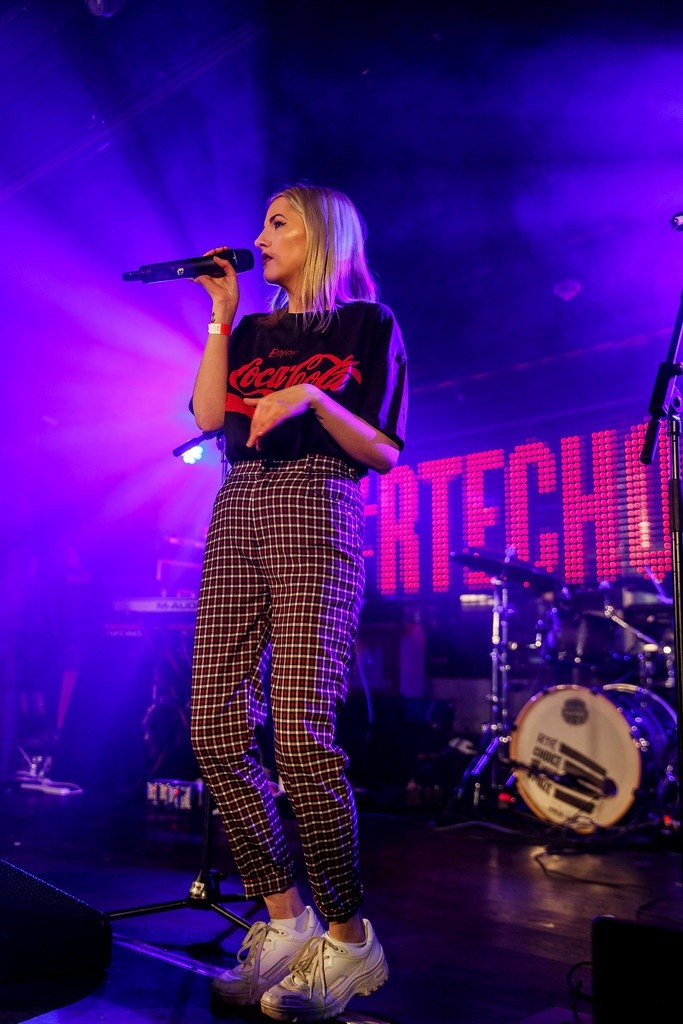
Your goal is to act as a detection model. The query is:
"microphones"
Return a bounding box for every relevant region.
[670,212,683,232]
[122,249,255,284]
[555,774,577,786]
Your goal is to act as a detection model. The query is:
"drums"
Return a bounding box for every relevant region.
[509,682,679,837]
[571,608,631,679]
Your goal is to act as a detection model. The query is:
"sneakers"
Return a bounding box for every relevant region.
[260,918,388,1022]
[210,905,326,1006]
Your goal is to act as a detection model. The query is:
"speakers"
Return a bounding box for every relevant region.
[0,860,112,1024]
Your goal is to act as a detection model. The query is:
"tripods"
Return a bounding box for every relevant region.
[104,785,265,934]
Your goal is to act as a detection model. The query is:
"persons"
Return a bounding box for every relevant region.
[189,184,408,1019]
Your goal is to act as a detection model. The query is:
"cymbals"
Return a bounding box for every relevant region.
[446,546,560,591]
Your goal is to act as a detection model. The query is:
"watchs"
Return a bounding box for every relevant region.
[207,322,230,337]
